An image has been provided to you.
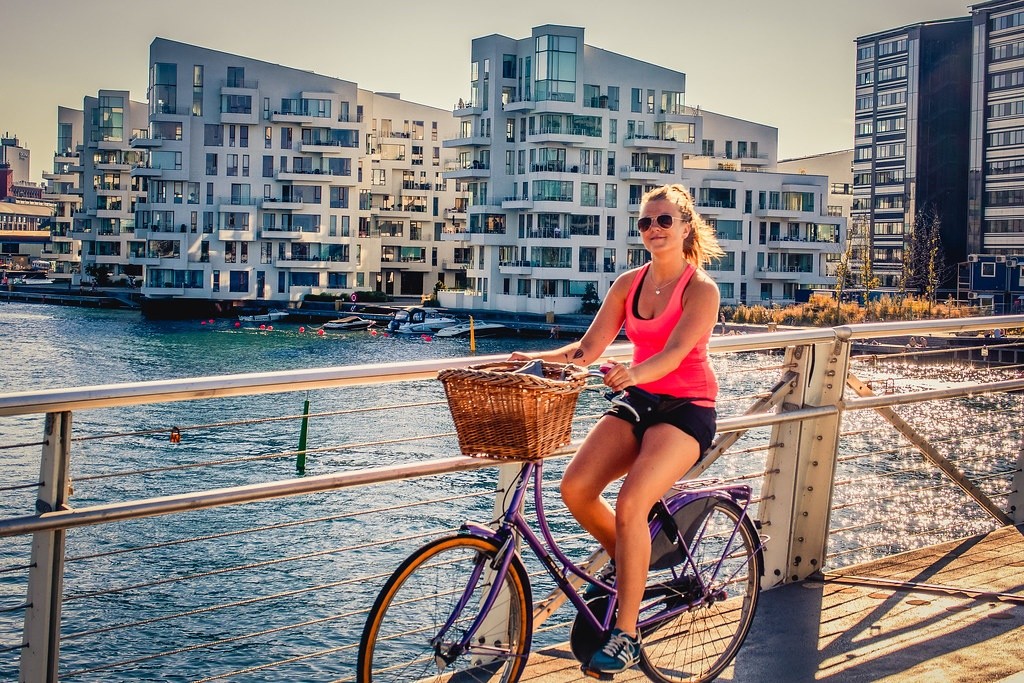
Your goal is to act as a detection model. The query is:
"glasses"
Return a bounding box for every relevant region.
[637,214,688,233]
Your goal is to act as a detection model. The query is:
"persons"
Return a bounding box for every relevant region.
[720,313,725,336]
[510,185,720,674]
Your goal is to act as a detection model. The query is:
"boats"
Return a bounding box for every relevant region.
[237,311,289,322]
[432,318,508,338]
[384,305,460,335]
[322,316,376,331]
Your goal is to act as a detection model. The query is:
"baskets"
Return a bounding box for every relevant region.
[438,359,590,461]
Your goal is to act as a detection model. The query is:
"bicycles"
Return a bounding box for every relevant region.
[356,357,772,683]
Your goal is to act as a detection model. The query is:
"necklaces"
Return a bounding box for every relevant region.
[650,259,684,294]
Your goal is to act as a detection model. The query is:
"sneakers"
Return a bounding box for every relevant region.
[588,628,641,674]
[582,559,617,601]
